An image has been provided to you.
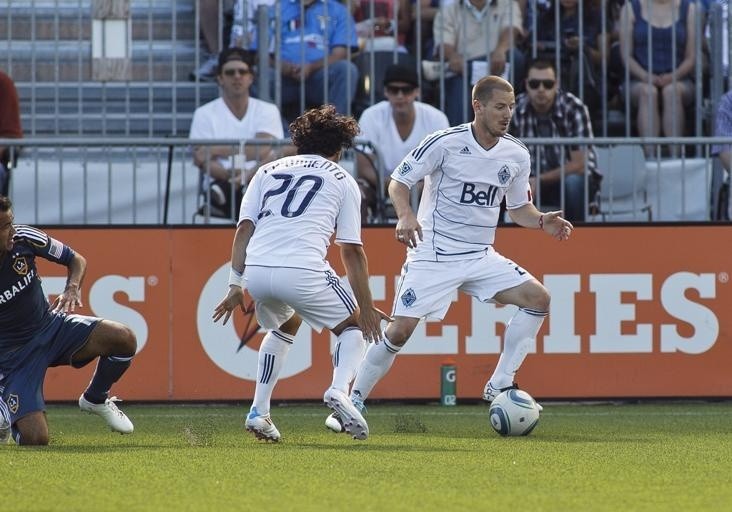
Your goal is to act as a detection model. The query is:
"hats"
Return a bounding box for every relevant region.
[384,64,418,86]
[219,48,250,65]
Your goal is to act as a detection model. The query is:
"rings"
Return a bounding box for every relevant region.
[398,234,404,239]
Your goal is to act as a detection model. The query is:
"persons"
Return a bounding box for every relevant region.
[1,72,24,199]
[210,103,395,442]
[324,75,573,435]
[0,196,138,447]
[190,0,732,227]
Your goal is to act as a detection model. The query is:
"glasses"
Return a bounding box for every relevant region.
[529,80,554,89]
[225,70,248,75]
[389,86,412,94]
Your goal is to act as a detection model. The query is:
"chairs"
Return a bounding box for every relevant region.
[192,167,224,223]
[595,144,653,221]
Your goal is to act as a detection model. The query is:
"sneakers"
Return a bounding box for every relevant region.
[79,393,134,433]
[245,407,280,440]
[190,54,220,81]
[324,388,369,439]
[483,381,543,411]
[211,184,226,206]
[422,60,455,81]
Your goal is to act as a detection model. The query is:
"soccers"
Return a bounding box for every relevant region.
[488,389,540,436]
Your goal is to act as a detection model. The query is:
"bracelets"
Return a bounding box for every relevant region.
[228,268,243,288]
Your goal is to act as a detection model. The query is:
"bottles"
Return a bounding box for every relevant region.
[440,355,457,406]
